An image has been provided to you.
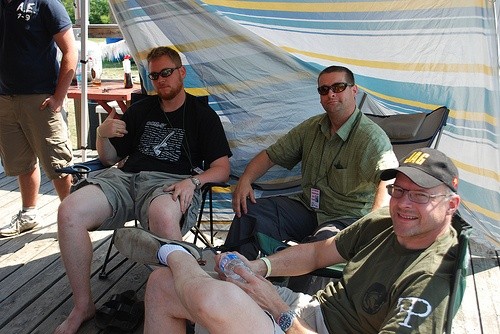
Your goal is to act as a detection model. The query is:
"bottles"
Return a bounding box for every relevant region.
[219,251,254,284]
[76,56,101,91]
[122,55,133,89]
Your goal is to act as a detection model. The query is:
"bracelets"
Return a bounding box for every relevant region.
[260,256,271,278]
[97,127,108,139]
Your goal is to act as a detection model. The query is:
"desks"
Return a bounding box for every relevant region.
[68,86,139,149]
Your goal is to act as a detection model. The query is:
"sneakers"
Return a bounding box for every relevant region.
[113,226,206,268]
[0,210,40,237]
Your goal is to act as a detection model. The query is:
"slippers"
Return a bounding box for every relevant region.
[95,290,144,334]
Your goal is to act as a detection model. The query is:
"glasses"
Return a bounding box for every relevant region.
[386,184,452,204]
[148,67,179,80]
[317,82,353,96]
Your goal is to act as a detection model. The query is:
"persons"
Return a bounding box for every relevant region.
[0,0,79,237]
[221,65,399,261]
[55,48,233,334]
[116,147,461,334]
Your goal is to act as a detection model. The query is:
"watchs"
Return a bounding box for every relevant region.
[189,175,201,190]
[277,309,298,332]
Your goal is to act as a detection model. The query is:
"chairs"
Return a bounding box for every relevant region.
[100,88,484,334]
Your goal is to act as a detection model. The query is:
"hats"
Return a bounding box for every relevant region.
[380,148,459,194]
